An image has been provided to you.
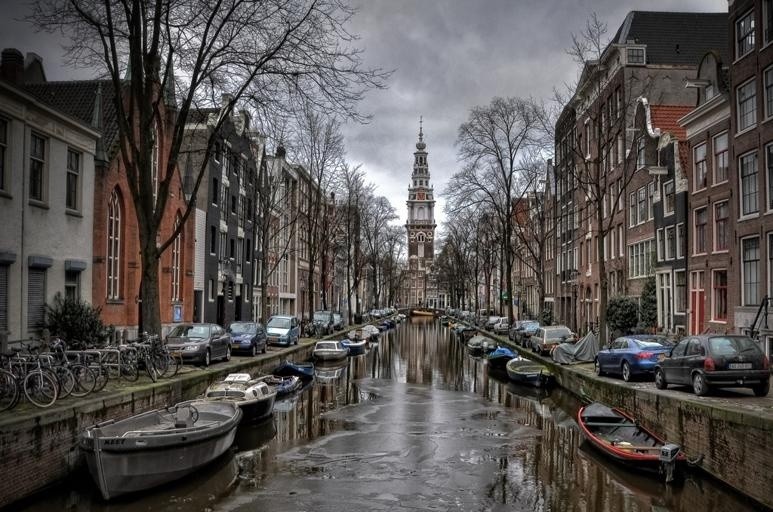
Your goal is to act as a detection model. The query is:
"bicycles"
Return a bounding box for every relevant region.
[0,332,183,412]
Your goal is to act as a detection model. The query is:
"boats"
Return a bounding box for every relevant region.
[441,315,556,387]
[412,310,434,316]
[577,402,687,483]
[312,311,407,360]
[79,360,317,501]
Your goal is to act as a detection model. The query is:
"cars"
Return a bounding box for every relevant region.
[369,305,396,319]
[595,334,770,397]
[164,314,301,367]
[448,307,577,357]
